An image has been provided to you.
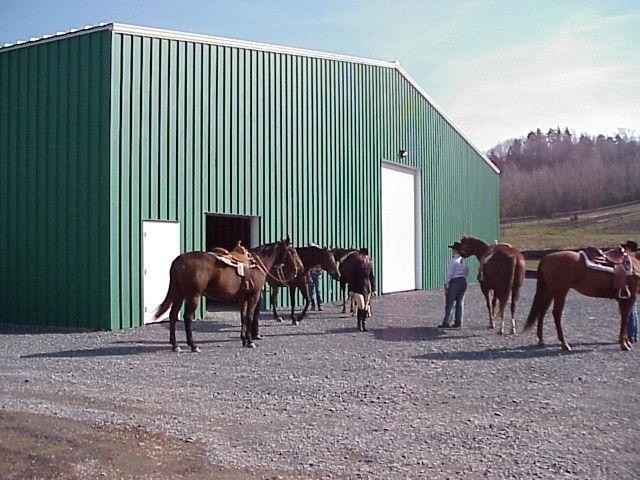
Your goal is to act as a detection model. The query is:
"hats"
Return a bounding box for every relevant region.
[359,248,369,255]
[447,241,467,255]
[620,240,640,251]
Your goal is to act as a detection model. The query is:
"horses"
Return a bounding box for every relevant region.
[266,246,341,326]
[459,237,526,334]
[151,236,304,353]
[520,251,640,351]
[329,249,376,317]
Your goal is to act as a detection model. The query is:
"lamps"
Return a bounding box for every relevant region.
[399,150,408,158]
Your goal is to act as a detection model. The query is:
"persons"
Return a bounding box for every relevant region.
[438,242,470,328]
[348,248,376,332]
[620,241,637,343]
[307,243,324,311]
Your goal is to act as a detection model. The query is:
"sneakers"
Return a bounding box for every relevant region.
[452,323,461,328]
[438,320,451,328]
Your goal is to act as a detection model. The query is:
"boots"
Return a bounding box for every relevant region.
[357,306,364,332]
[362,310,370,332]
